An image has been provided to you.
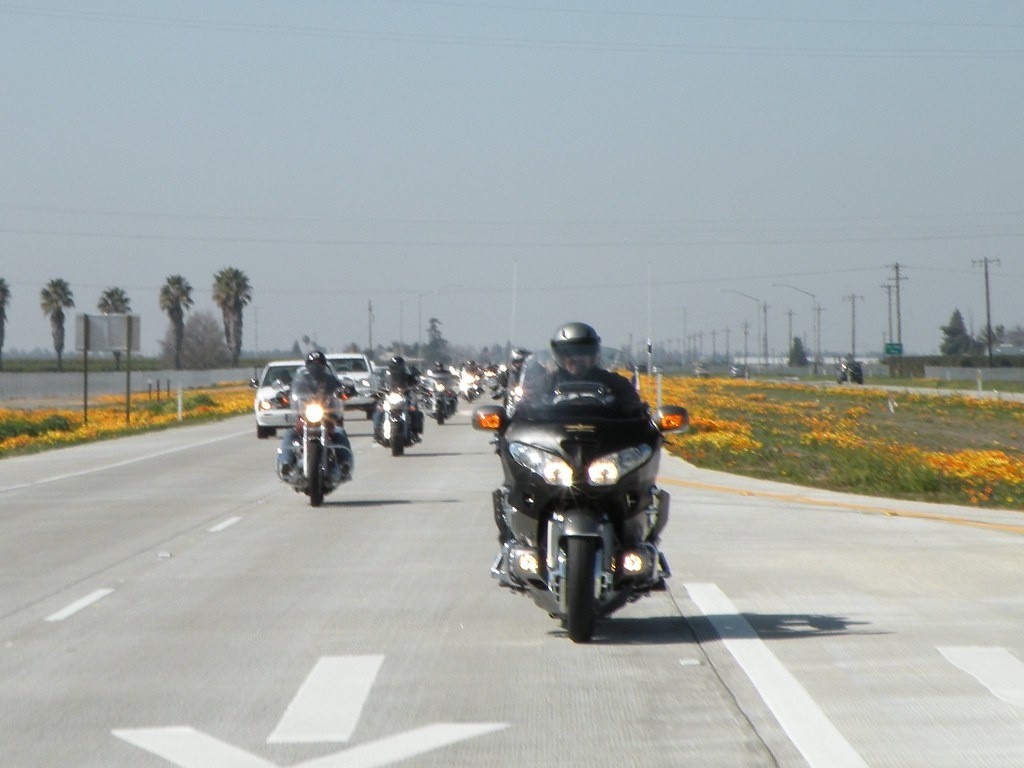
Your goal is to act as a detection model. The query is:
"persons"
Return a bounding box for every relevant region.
[492,347,548,408]
[423,361,459,413]
[280,351,353,477]
[371,357,420,441]
[281,370,292,382]
[492,322,667,591]
[459,360,499,378]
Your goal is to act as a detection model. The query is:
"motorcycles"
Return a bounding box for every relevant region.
[360,367,430,457]
[460,367,485,404]
[833,362,864,385]
[487,362,521,456]
[471,344,692,644]
[418,366,461,425]
[270,362,356,507]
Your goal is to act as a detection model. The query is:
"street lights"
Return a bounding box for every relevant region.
[772,283,817,373]
[720,289,760,363]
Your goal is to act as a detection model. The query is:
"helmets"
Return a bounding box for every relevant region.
[434,361,443,372]
[305,351,326,375]
[388,357,406,371]
[509,347,529,374]
[550,322,601,376]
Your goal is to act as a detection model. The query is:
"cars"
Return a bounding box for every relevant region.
[325,354,380,420]
[249,360,345,439]
